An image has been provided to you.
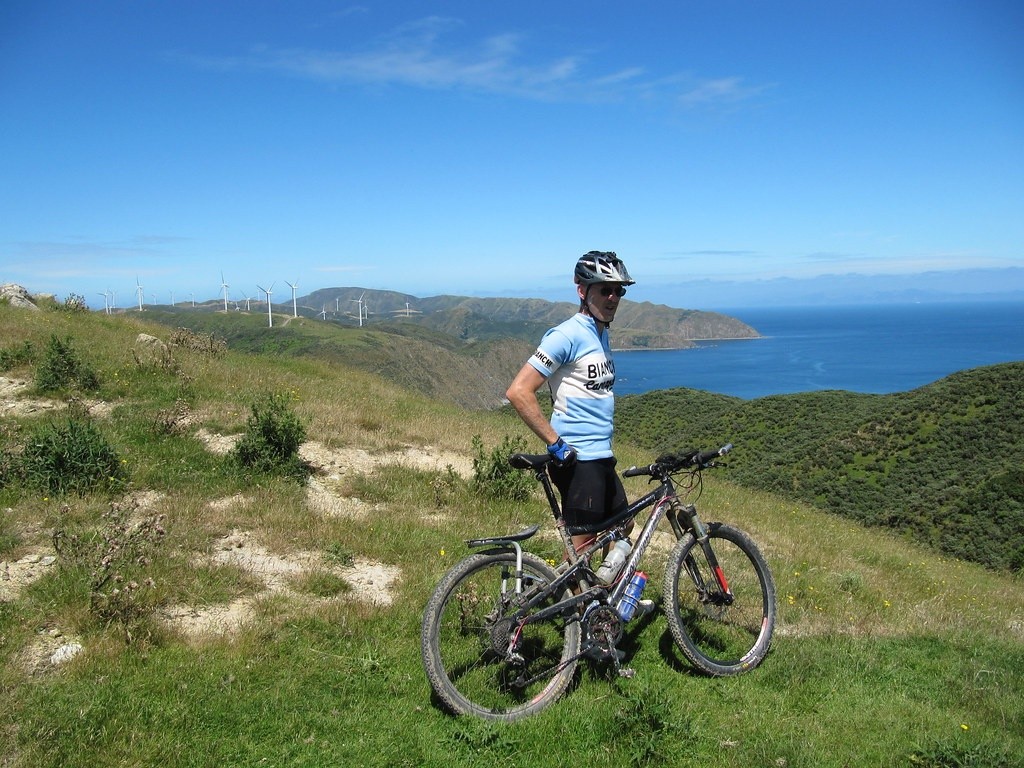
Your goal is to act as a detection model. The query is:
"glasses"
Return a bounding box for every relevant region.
[592,286,627,297]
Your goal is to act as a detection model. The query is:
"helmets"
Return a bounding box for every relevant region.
[574,250,635,284]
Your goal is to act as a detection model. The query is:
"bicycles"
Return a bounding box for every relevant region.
[421,443,777,722]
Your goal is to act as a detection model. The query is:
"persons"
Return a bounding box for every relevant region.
[505,252,654,661]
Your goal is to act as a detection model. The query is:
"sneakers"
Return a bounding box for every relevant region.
[589,648,625,661]
[632,600,654,617]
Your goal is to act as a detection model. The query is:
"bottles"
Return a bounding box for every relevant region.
[595,537,632,584]
[616,570,647,623]
